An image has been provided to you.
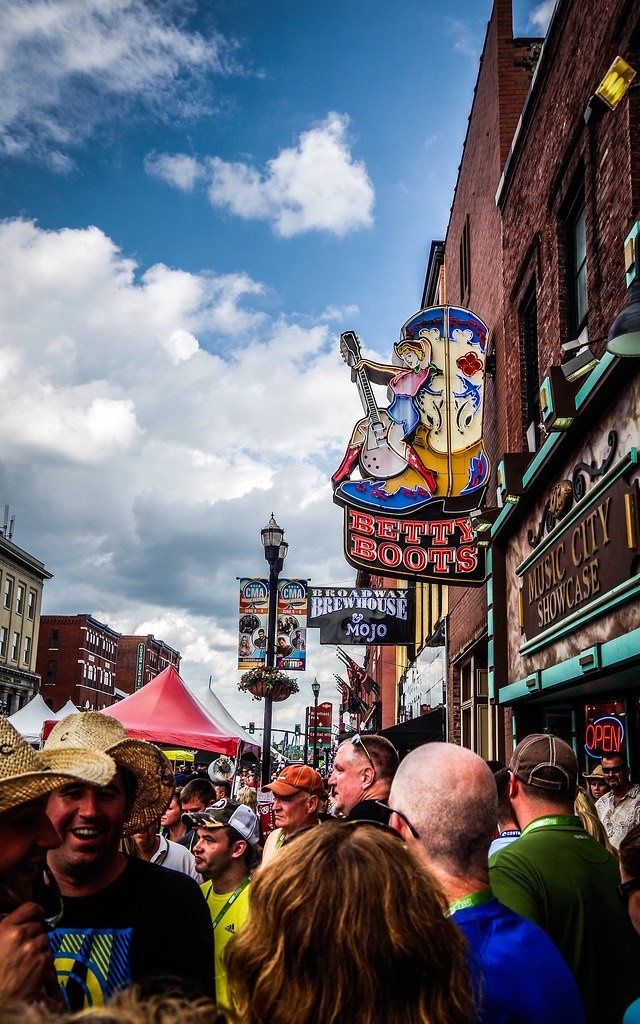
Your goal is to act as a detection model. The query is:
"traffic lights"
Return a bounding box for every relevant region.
[249,722,255,733]
[295,724,301,736]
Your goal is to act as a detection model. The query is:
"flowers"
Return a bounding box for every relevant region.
[238,665,300,702]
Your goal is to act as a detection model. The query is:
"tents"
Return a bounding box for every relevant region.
[92,664,289,798]
[6,694,82,745]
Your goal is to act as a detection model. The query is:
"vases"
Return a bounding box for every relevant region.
[249,677,290,702]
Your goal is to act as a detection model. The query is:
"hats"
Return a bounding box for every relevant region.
[44,712,177,840]
[582,765,606,785]
[260,763,325,802]
[0,715,117,811]
[507,733,578,790]
[180,797,260,848]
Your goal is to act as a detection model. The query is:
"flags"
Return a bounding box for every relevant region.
[338,656,377,714]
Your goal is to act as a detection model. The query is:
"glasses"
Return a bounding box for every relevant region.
[351,733,376,773]
[373,797,420,840]
[280,818,408,847]
[242,771,248,773]
[0,860,66,927]
[617,877,640,908]
[602,763,628,774]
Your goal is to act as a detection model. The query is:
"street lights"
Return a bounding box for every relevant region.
[261,511,290,787]
[311,678,321,770]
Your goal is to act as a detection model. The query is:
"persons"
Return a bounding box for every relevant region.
[278,617,297,635]
[277,637,292,657]
[239,636,253,657]
[0,711,640,1024]
[292,630,305,650]
[254,629,267,648]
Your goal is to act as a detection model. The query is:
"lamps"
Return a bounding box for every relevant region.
[539,365,576,431]
[605,274,640,358]
[467,454,523,549]
[560,336,607,382]
